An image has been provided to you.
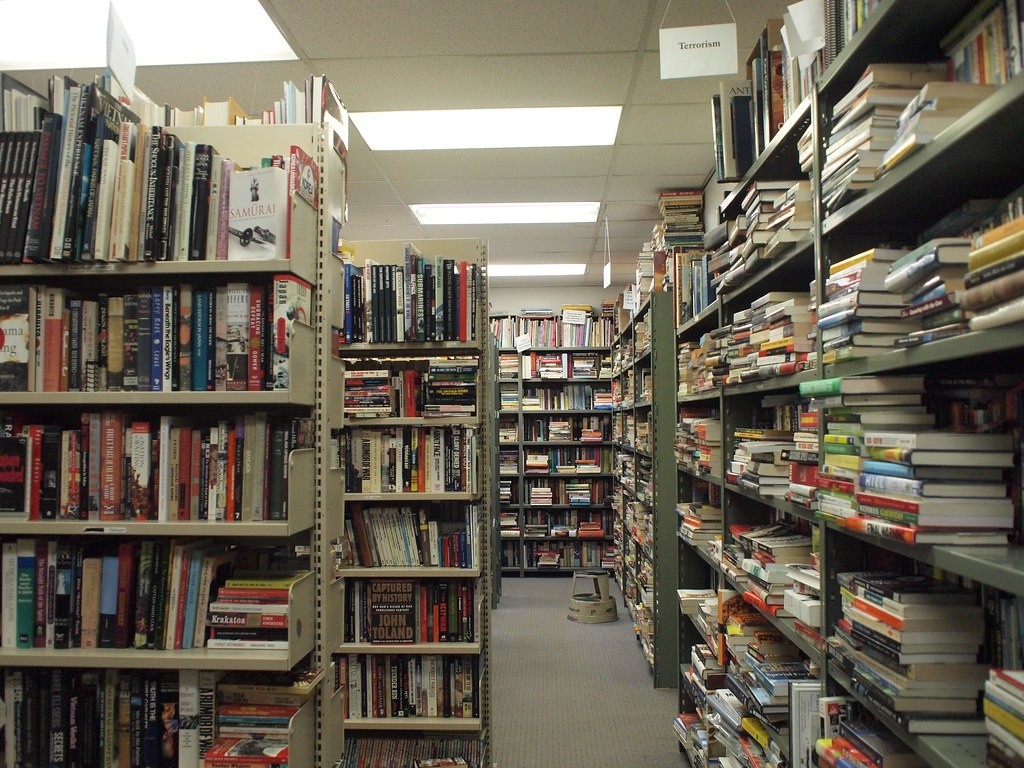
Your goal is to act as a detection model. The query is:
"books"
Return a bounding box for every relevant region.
[619,0,1024,766]
[0,71,346,768]
[488,300,618,569]
[346,249,484,765]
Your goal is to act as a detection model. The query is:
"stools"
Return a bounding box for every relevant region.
[567,569,619,624]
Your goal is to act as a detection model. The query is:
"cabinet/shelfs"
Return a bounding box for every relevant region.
[488,0,1024,768]
[331,238,496,768]
[1,121,347,768]
